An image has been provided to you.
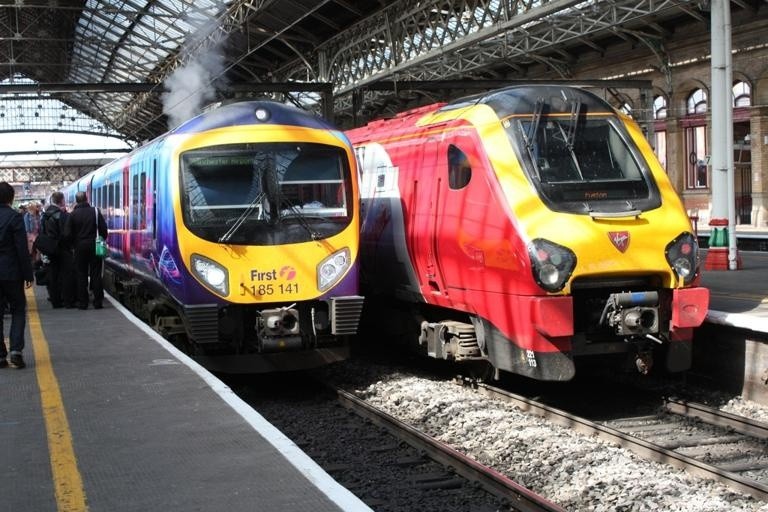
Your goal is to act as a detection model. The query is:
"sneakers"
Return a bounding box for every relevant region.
[10,354,27,369]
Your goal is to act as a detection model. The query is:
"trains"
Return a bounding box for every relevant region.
[49,99,366,380]
[343,80,713,406]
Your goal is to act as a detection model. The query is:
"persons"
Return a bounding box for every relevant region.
[0,181,34,367]
[19,191,108,309]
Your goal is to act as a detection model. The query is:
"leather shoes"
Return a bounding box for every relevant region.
[46,295,106,310]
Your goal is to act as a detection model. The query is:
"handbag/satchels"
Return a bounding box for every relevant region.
[34,211,65,257]
[35,267,49,286]
[92,205,109,258]
